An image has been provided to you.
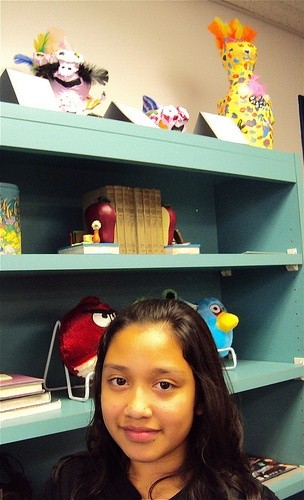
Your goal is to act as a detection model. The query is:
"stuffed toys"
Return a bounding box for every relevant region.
[190,297,239,358]
[58,297,119,380]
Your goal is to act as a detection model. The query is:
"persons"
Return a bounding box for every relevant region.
[37,297,280,500]
[0,453,33,500]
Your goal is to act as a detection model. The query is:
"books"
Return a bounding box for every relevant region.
[0,373,62,422]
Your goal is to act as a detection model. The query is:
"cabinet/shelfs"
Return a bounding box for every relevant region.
[0,101,304,500]
